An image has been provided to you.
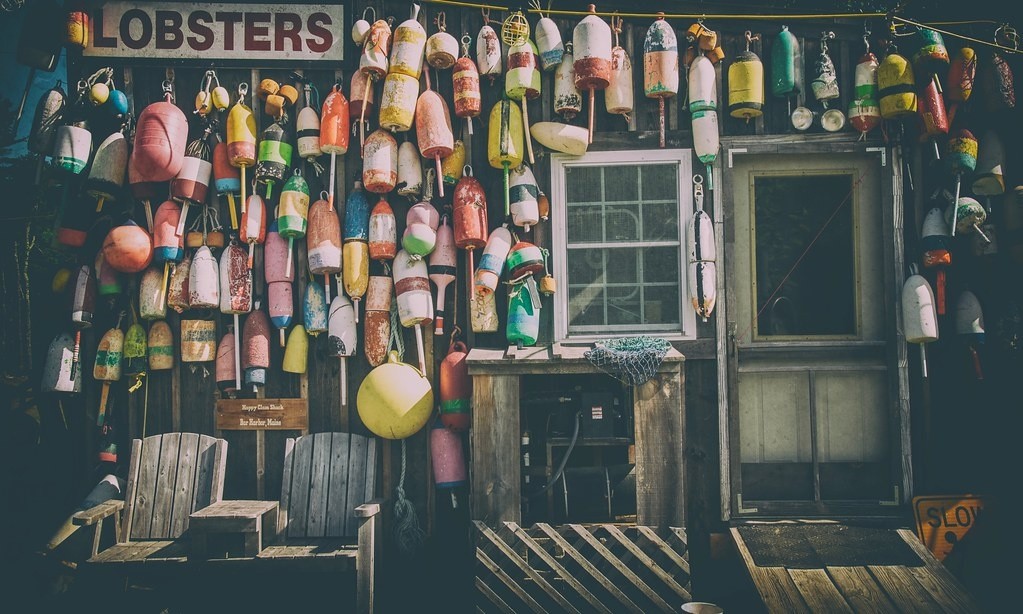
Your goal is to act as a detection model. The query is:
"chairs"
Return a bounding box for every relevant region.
[72,432,229,614]
[256,431,391,614]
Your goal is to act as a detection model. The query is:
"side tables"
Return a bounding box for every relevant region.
[188,500,279,559]
